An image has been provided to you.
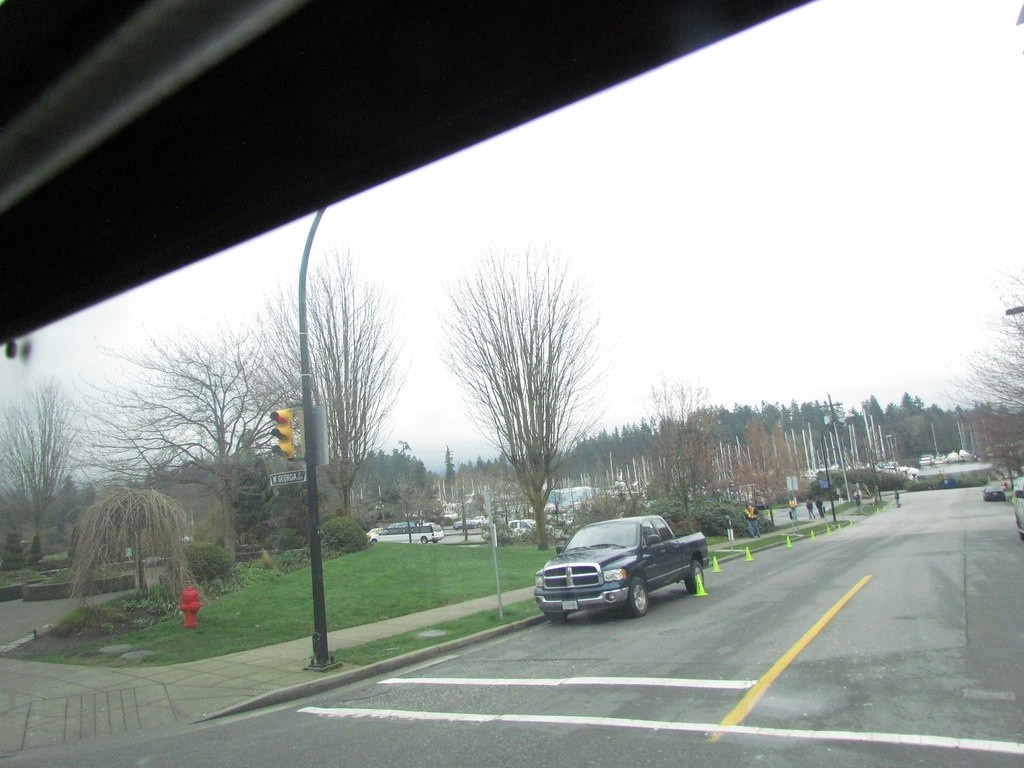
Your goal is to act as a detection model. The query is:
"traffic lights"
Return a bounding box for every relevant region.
[268,407,297,462]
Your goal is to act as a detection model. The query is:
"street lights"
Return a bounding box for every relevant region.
[820,413,856,523]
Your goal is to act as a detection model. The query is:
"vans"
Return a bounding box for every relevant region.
[372,523,445,544]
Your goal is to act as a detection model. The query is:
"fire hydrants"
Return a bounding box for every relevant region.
[179,585,202,629]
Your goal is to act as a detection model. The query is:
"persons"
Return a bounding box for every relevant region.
[894,490,901,508]
[789,498,796,518]
[806,498,815,518]
[744,505,761,538]
[815,498,824,519]
[854,487,862,508]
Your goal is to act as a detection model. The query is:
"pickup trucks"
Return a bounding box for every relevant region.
[533,515,710,623]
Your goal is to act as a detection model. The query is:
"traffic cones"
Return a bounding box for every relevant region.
[744,547,754,562]
[786,536,793,548]
[876,508,881,514]
[849,520,854,527]
[693,571,708,600]
[826,526,832,536]
[837,523,842,532]
[712,556,722,573]
[810,530,816,541]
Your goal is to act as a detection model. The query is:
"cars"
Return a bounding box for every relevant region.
[984,485,1008,503]
[1012,478,1024,538]
[454,515,553,537]
